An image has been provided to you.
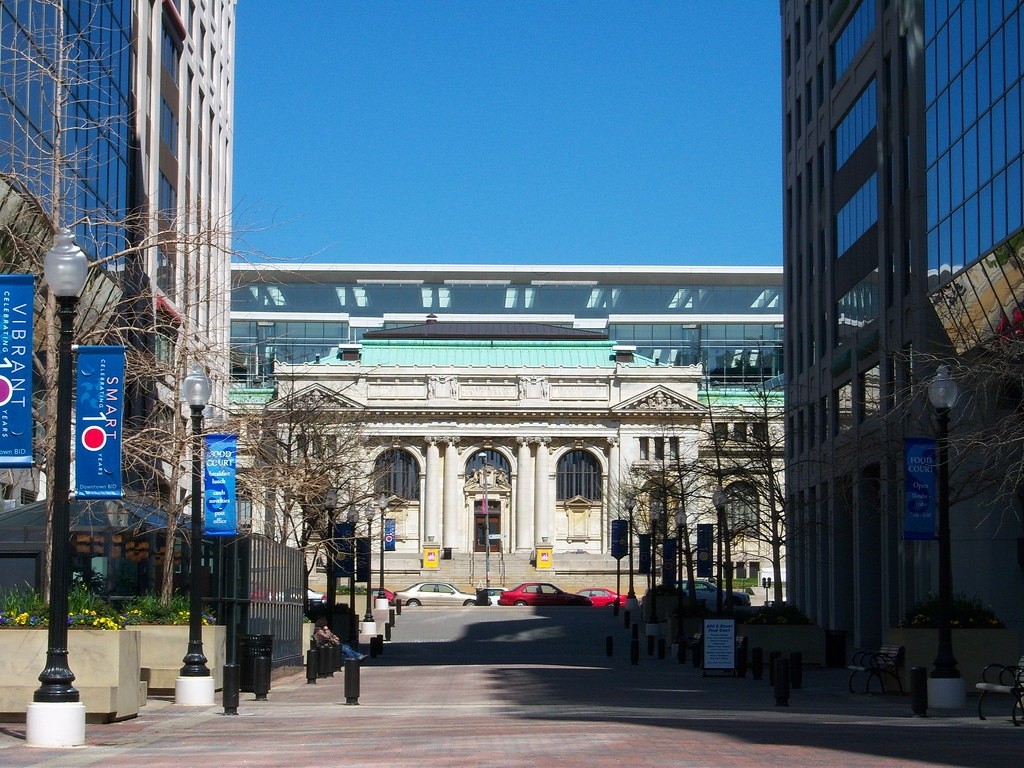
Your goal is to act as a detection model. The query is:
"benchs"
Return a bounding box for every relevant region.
[976,655,1024,727]
[847,644,905,697]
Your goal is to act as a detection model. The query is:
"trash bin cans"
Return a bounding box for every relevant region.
[443,548,452,559]
[237,633,273,692]
[475,587,488,606]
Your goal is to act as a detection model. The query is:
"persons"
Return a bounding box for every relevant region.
[313,617,369,664]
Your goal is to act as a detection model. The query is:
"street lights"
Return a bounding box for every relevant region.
[33,224,91,701]
[930,362,961,679]
[178,361,213,677]
[363,501,375,623]
[346,502,359,643]
[377,493,387,599]
[323,489,339,632]
[675,506,687,643]
[649,505,660,621]
[628,499,635,599]
[713,486,728,619]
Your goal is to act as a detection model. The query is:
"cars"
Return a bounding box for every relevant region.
[640,579,752,610]
[498,582,593,606]
[371,587,394,605]
[392,582,493,607]
[268,586,328,605]
[474,586,511,606]
[574,586,637,608]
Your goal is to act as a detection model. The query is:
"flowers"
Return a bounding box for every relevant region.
[745,601,816,626]
[645,585,687,596]
[116,588,217,625]
[898,590,1010,632]
[0,576,129,630]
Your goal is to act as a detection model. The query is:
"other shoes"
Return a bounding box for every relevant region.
[359,655,367,660]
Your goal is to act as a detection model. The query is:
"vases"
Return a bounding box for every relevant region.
[885,627,1019,695]
[0,631,147,725]
[642,596,689,621]
[124,626,227,696]
[737,623,825,669]
